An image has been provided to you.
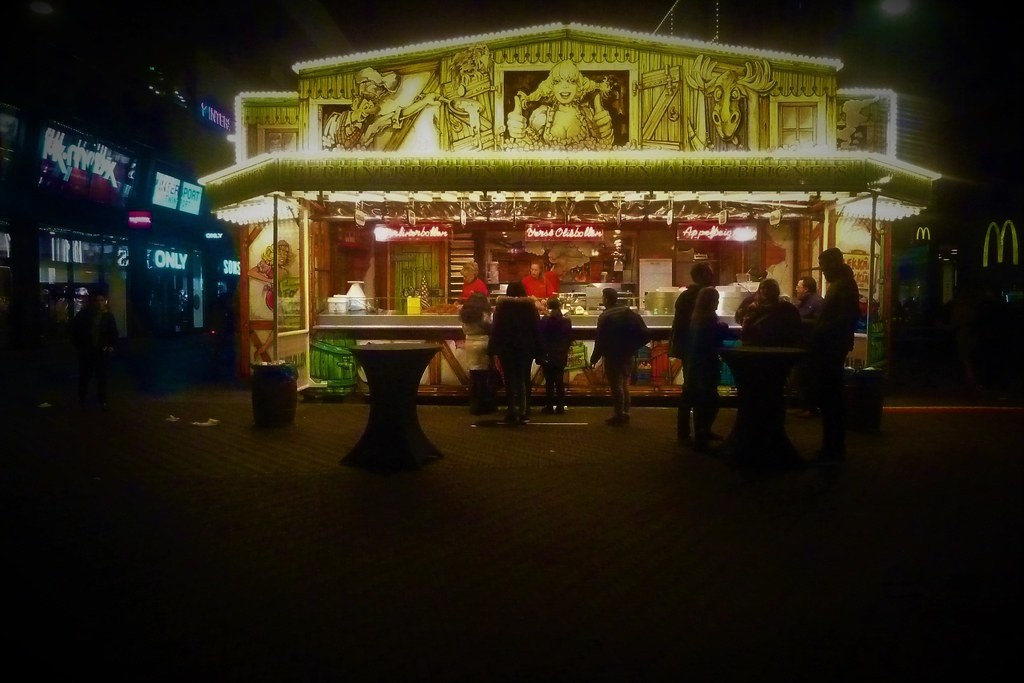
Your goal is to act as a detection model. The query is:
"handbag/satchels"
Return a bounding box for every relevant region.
[468,366,504,415]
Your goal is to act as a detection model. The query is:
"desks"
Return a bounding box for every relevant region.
[718,347,807,468]
[350,344,445,473]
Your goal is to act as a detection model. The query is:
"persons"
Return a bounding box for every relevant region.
[76,291,119,412]
[458,261,574,423]
[950,288,983,379]
[590,288,634,426]
[671,248,859,468]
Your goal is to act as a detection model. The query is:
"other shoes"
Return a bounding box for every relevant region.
[519,415,530,423]
[623,415,630,424]
[605,417,623,428]
[707,433,725,440]
[792,409,819,419]
[503,415,516,423]
[541,407,554,415]
[555,406,566,415]
[677,434,693,447]
[693,442,722,455]
[806,449,847,467]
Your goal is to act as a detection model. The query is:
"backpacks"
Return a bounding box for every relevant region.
[625,307,652,347]
